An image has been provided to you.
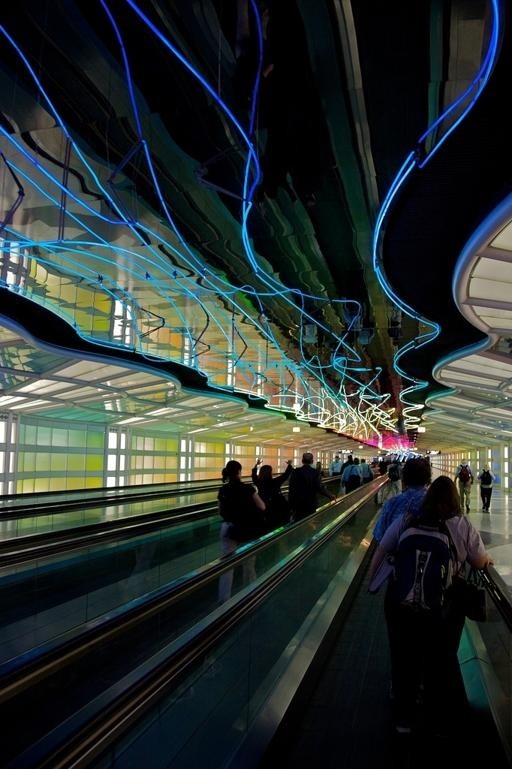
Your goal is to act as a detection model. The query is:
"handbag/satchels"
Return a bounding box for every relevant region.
[465,581,486,622]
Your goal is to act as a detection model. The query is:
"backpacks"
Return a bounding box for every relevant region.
[481,470,491,484]
[389,515,452,610]
[460,465,468,482]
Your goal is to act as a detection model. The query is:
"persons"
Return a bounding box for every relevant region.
[368,455,489,733]
[478,464,495,513]
[329,454,374,524]
[217,453,337,589]
